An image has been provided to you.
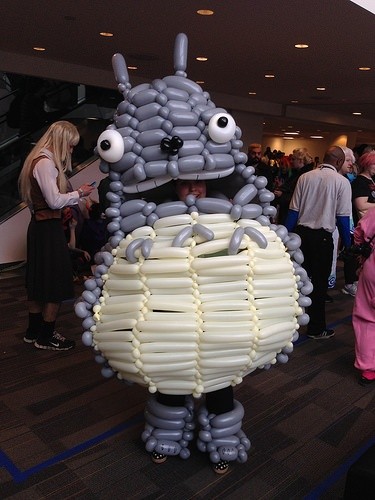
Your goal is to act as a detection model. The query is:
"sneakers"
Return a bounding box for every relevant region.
[34,329,75,350]
[24,328,41,343]
[341,284,357,297]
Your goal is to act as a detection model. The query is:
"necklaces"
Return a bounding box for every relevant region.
[318,165,334,170]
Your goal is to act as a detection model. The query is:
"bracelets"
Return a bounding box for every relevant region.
[79,188,84,196]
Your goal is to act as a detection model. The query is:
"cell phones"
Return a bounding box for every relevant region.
[89,181,96,186]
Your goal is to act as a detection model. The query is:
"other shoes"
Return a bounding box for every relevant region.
[306,329,335,339]
[211,459,229,474]
[359,375,375,386]
[152,452,168,463]
[325,295,333,303]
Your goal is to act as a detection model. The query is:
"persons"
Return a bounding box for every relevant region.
[246,143,321,225]
[326,146,375,380]
[143,179,233,473]
[285,146,352,339]
[64,206,93,280]
[89,176,112,240]
[18,121,94,351]
